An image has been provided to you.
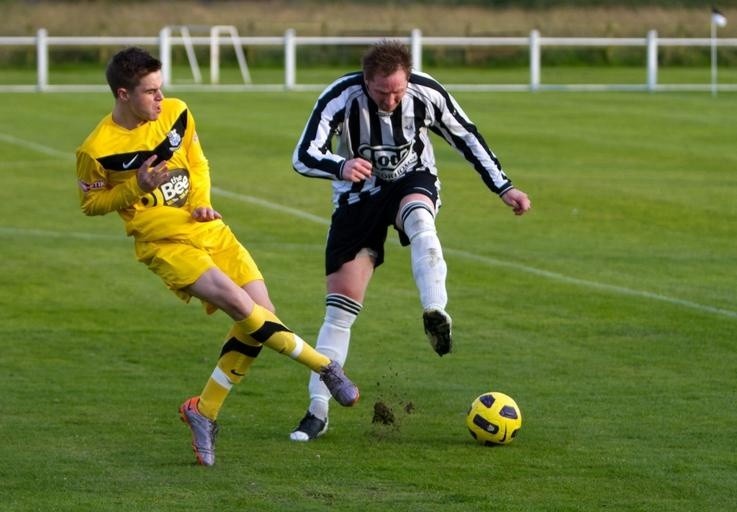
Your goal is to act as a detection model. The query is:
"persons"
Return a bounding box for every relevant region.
[286,36,533,443]
[71,49,360,468]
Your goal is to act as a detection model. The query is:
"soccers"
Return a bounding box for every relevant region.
[466,392,522,446]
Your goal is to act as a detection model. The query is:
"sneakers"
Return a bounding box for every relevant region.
[420,306,452,357]
[289,411,330,443]
[176,396,218,466]
[319,360,360,407]
